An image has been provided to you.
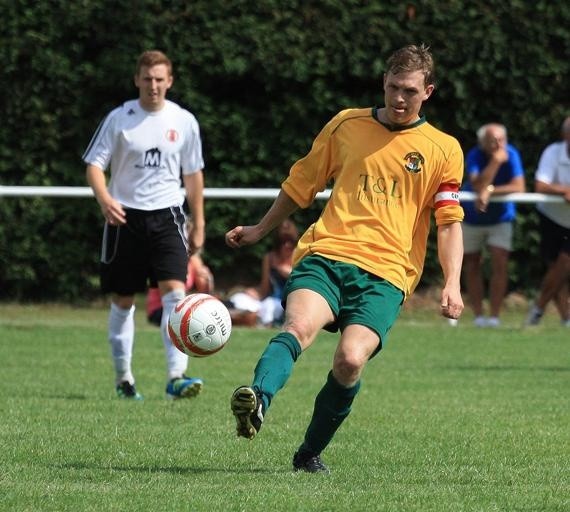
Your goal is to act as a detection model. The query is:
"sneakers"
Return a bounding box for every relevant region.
[229,383,268,441]
[115,381,142,401]
[471,314,501,329]
[164,374,205,401]
[290,450,331,475]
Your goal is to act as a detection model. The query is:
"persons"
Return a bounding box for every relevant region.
[227,219,298,329]
[459,120,528,327]
[224,41,467,474]
[78,48,205,397]
[143,214,216,332]
[527,115,570,332]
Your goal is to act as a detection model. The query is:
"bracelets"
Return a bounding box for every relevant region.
[485,184,495,193]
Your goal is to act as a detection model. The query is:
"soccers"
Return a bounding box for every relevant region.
[168,293,232,357]
